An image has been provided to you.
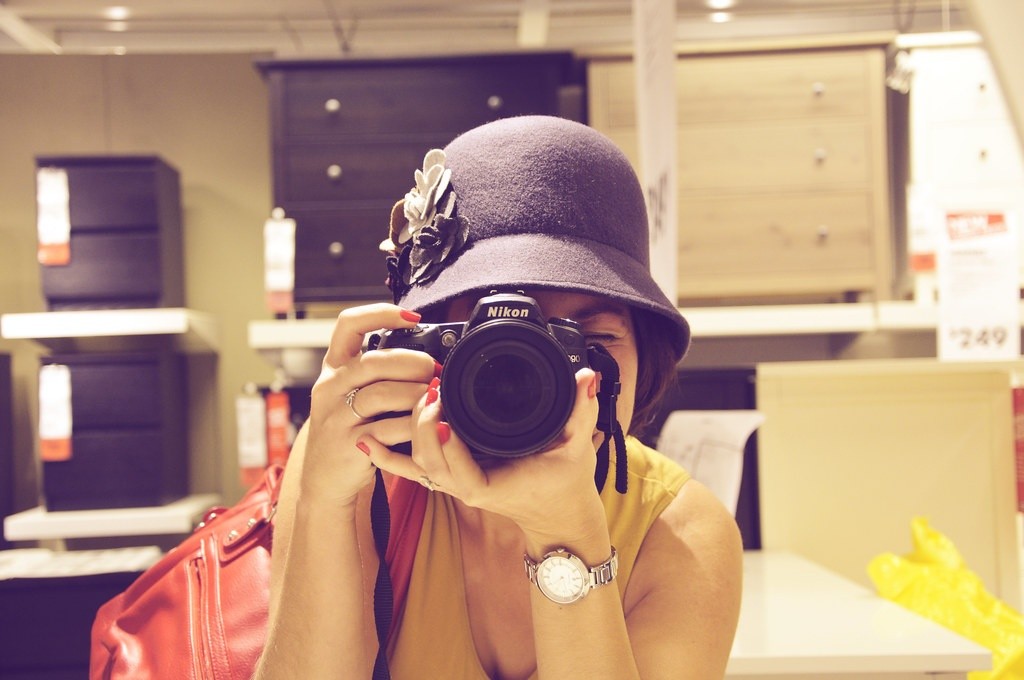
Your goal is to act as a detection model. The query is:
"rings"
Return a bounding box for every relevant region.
[346,388,369,420]
[420,476,441,491]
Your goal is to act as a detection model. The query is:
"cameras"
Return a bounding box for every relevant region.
[367,290,586,458]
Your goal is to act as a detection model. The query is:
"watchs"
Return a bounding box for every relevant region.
[523,544,618,606]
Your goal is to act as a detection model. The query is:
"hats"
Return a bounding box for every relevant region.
[379,115,692,365]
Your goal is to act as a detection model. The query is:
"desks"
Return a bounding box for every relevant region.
[722,549,994,680]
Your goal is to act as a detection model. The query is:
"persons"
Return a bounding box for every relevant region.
[254,114,744,680]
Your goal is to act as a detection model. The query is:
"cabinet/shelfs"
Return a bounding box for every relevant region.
[254,50,588,321]
[34,155,185,310]
[752,359,1024,614]
[569,29,899,305]
[37,350,188,513]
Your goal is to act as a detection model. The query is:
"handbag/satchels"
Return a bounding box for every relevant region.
[83,464,286,680]
